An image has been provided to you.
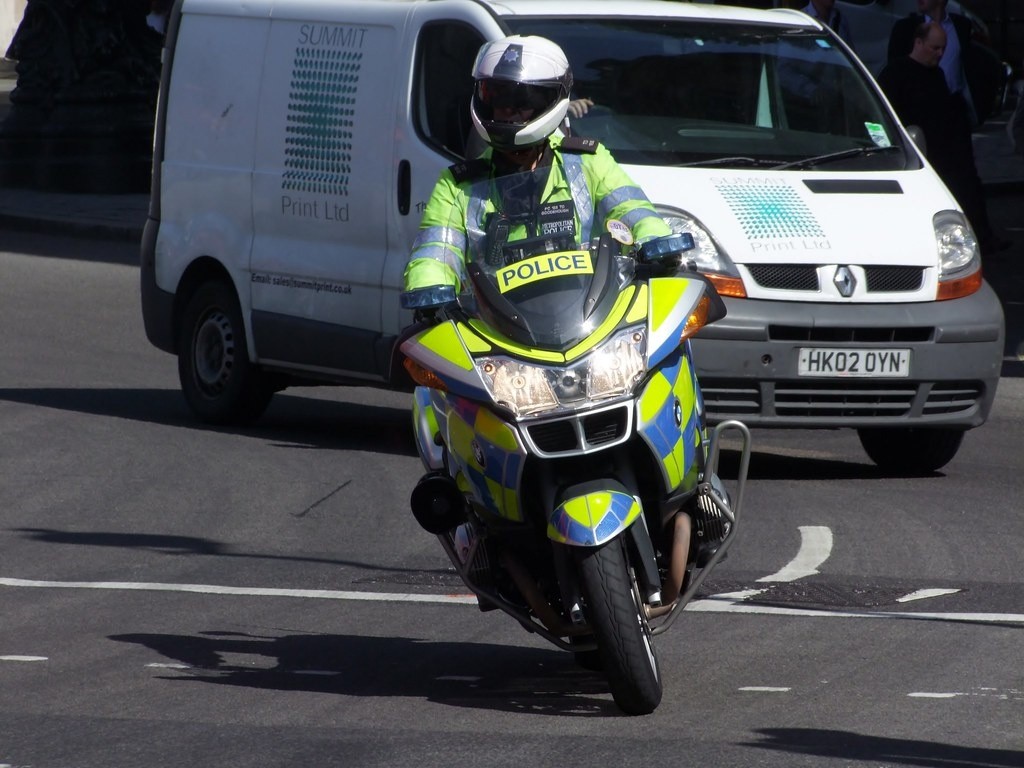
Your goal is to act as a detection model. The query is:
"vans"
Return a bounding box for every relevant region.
[136,0,1006,480]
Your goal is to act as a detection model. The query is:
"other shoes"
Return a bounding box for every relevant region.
[982,237,1015,257]
[695,540,726,568]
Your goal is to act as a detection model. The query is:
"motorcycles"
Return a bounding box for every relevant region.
[389,230,754,717]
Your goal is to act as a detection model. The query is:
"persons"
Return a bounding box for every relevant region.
[775,0,1016,255]
[399,32,698,325]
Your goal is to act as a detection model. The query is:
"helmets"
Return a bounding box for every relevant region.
[470,33,573,152]
